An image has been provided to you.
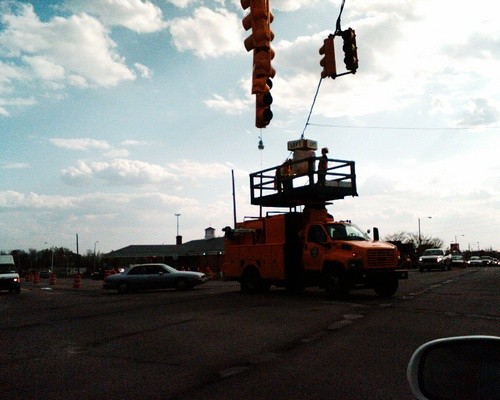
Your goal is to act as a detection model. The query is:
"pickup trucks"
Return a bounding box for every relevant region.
[419,249,452,272]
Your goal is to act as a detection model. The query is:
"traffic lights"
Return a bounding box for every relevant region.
[319,38,336,80]
[240,1,254,51]
[342,29,358,70]
[257,78,272,127]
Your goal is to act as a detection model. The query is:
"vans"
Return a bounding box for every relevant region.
[1,255,21,295]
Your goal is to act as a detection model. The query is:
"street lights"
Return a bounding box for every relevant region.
[418,217,431,244]
[455,235,464,243]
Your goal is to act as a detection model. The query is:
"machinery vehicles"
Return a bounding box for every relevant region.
[220,157,409,298]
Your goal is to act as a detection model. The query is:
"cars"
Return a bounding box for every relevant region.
[452,256,500,267]
[102,263,207,294]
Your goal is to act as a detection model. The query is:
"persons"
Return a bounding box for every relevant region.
[318,147,328,183]
